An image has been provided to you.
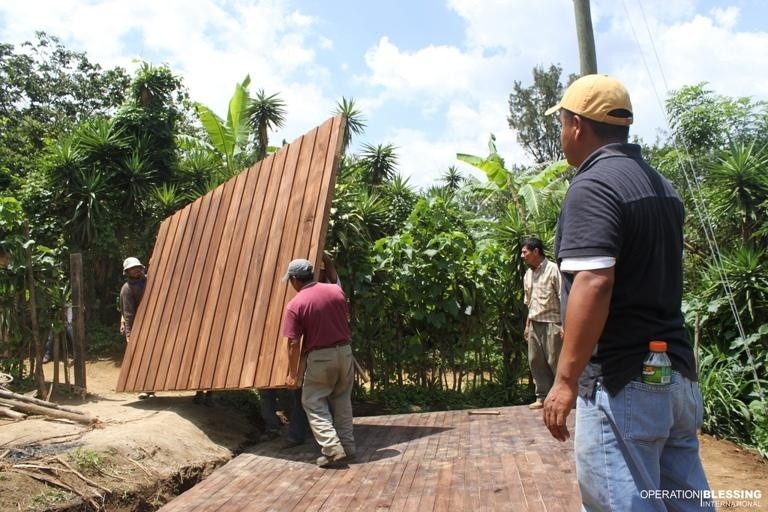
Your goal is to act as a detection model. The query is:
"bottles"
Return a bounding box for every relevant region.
[642,341,673,386]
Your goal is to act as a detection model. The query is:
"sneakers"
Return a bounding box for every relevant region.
[528,401,543,409]
[316,453,346,467]
[194,391,214,406]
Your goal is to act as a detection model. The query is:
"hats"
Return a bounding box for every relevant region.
[544,74,633,125]
[282,258,315,283]
[122,256,146,275]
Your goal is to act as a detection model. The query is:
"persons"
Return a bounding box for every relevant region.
[520,238,576,408]
[42,300,73,363]
[192,390,215,407]
[119,256,153,399]
[542,74,716,512]
[250,384,288,443]
[285,252,342,449]
[283,260,356,467]
